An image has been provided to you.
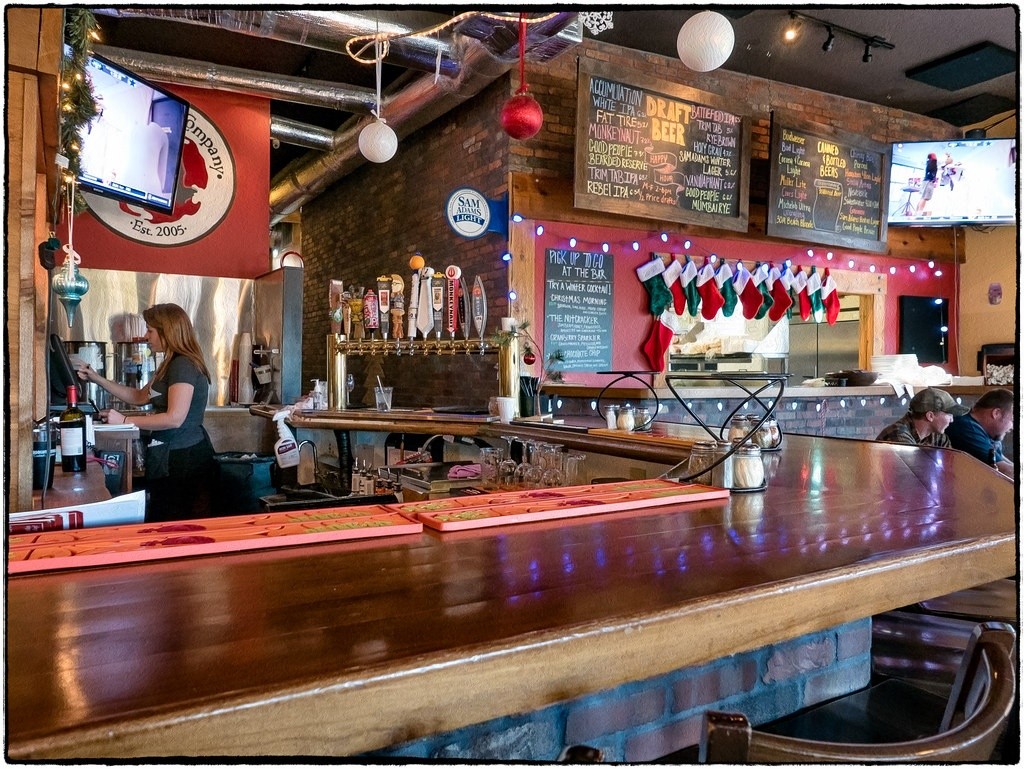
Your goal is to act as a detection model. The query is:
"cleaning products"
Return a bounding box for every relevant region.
[272,410,301,469]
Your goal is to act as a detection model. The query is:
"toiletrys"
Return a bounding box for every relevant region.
[310,379,323,410]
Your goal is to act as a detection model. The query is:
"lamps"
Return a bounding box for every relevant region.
[783,10,896,63]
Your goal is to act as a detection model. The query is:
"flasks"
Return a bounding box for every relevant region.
[33,429,56,488]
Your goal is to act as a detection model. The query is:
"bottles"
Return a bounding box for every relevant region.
[350,467,404,504]
[728,414,780,448]
[605,405,652,431]
[689,437,765,489]
[59,386,88,474]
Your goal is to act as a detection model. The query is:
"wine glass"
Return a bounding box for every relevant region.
[499,435,586,489]
[346,375,355,405]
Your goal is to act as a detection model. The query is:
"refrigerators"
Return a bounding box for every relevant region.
[790,321,857,385]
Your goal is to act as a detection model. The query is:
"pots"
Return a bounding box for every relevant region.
[825,370,882,386]
[802,377,848,387]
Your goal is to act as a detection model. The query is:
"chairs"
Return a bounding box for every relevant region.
[698,621,1017,764]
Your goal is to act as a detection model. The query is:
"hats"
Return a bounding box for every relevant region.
[910,385,970,415]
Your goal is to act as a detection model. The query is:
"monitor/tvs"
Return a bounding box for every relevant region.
[64,36,189,215]
[886,137,1017,228]
[49,334,80,394]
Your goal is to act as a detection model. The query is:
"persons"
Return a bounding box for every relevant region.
[76,303,218,522]
[914,152,937,217]
[875,387,970,449]
[946,389,1015,481]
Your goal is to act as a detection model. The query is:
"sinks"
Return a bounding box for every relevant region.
[262,489,337,505]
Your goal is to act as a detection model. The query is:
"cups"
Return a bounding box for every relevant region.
[480,447,504,488]
[254,364,271,384]
[238,333,252,402]
[374,387,393,412]
[496,396,514,423]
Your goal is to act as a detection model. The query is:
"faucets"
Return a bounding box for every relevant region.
[299,440,335,489]
[134,364,148,383]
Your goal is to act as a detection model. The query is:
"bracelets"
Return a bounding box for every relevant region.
[123,416,127,423]
